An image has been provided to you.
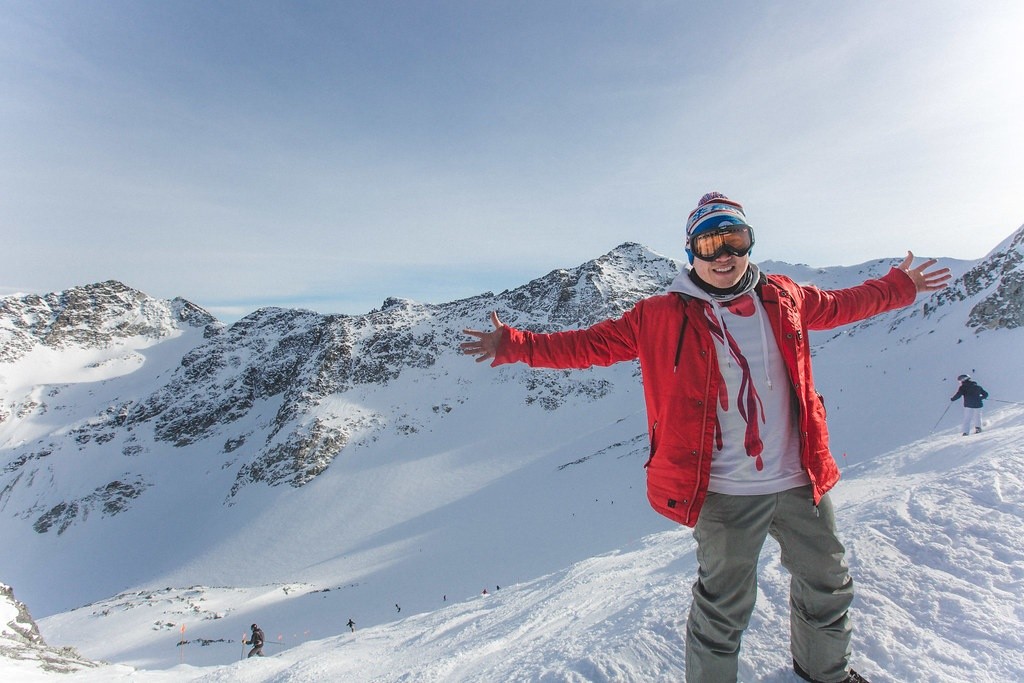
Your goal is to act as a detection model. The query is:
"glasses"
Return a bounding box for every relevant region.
[689,226,756,260]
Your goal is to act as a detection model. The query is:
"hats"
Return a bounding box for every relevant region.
[685,192,753,264]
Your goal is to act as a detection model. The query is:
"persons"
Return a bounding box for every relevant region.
[497,585,501,590]
[482,589,488,594]
[242,624,265,658]
[460,192,951,683]
[346,619,356,632]
[396,604,401,612]
[951,375,988,436]
[444,595,446,601]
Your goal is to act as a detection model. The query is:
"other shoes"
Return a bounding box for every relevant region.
[963,433,969,436]
[793,658,867,683]
[976,427,982,433]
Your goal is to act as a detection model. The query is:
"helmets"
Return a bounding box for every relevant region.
[958,375,970,383]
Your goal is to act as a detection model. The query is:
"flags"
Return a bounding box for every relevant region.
[242,632,246,644]
[179,624,186,633]
[278,632,283,640]
